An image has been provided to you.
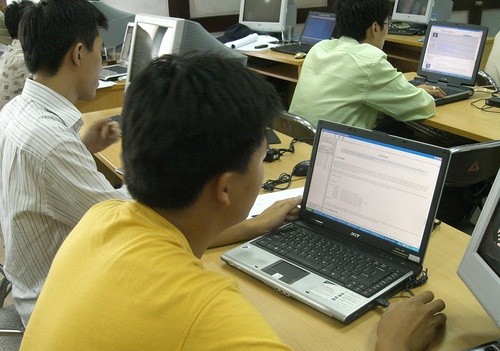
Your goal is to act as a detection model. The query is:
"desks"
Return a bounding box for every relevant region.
[76,34,500,351]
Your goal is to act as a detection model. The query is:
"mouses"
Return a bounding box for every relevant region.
[292,159,310,176]
[264,149,280,162]
[295,52,306,59]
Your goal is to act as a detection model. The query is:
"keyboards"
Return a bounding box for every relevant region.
[387,27,419,35]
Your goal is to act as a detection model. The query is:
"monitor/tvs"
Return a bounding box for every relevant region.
[392,0,454,25]
[238,0,297,36]
[456,168,500,326]
[124,13,248,92]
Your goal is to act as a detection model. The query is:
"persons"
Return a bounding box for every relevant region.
[0,0,37,112]
[19,50,447,351]
[0,0,133,330]
[289,0,446,132]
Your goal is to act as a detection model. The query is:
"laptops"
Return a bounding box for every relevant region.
[271,10,338,54]
[220,119,450,325]
[100,23,134,80]
[407,22,488,105]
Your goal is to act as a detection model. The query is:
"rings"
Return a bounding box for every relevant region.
[433,86,435,89]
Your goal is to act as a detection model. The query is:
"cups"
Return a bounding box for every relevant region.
[104,46,116,66]
[281,25,295,45]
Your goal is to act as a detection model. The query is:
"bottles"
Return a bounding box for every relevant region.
[430,14,437,21]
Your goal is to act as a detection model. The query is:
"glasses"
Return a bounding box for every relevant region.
[381,20,393,28]
